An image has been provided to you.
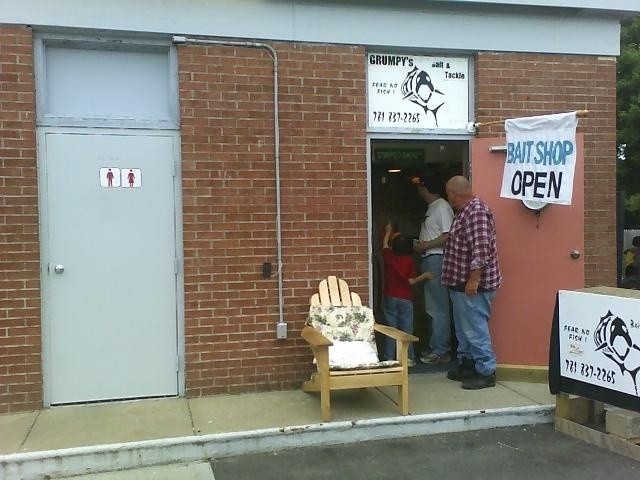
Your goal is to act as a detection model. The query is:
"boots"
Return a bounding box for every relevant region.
[447,359,497,389]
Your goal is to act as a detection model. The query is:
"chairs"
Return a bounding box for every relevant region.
[299,272,421,425]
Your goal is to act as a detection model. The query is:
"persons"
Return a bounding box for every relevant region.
[620,236,640,290]
[380,169,504,389]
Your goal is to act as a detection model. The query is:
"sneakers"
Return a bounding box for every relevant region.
[420,349,452,365]
[407,359,416,367]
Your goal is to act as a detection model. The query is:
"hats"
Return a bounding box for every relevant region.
[412,169,442,193]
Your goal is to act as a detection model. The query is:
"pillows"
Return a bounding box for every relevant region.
[326,338,380,369]
